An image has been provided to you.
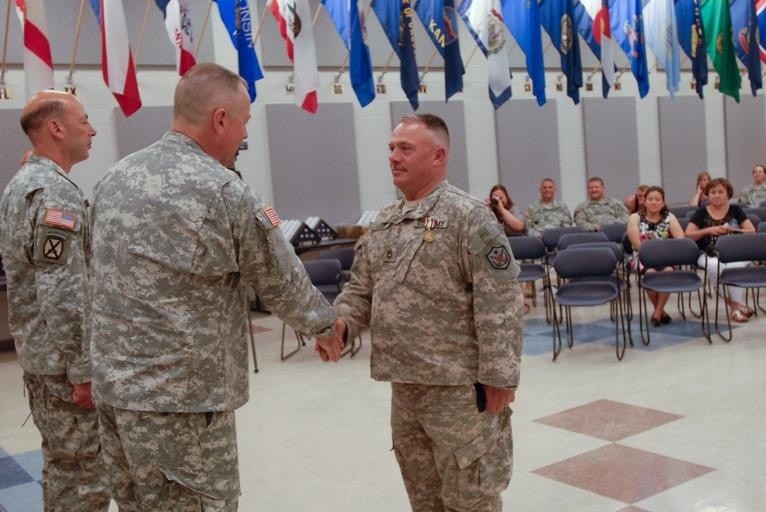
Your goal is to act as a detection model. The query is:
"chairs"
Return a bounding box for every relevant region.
[506,237,552,324]
[638,237,712,345]
[553,247,626,360]
[716,232,766,342]
[280,259,362,360]
[319,247,354,271]
[522,201,766,252]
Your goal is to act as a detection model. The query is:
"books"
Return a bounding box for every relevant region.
[275,215,341,251]
[355,207,383,228]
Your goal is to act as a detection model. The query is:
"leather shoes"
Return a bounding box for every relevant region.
[650,310,662,328]
[661,315,673,326]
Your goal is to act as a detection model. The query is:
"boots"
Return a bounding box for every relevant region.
[522,280,535,298]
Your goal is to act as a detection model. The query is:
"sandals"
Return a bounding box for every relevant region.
[731,309,750,323]
[737,304,754,319]
[524,303,530,315]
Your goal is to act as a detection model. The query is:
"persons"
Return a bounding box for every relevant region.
[484,184,527,237]
[85,64,343,510]
[625,187,684,327]
[738,165,766,208]
[574,178,629,229]
[690,172,712,207]
[0,91,110,512]
[626,183,651,213]
[526,179,571,235]
[684,178,755,322]
[315,113,525,512]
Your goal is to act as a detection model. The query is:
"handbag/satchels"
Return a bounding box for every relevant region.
[706,228,737,258]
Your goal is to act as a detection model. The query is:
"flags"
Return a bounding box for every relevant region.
[91,0,142,119]
[752,1,766,64]
[270,0,319,113]
[674,0,709,101]
[158,0,195,75]
[640,1,681,101]
[17,0,52,108]
[606,0,650,99]
[369,2,420,110]
[536,1,581,107]
[499,1,546,108]
[570,0,617,100]
[727,0,761,98]
[325,1,375,108]
[699,1,742,104]
[413,1,465,103]
[215,1,265,105]
[458,1,512,110]
